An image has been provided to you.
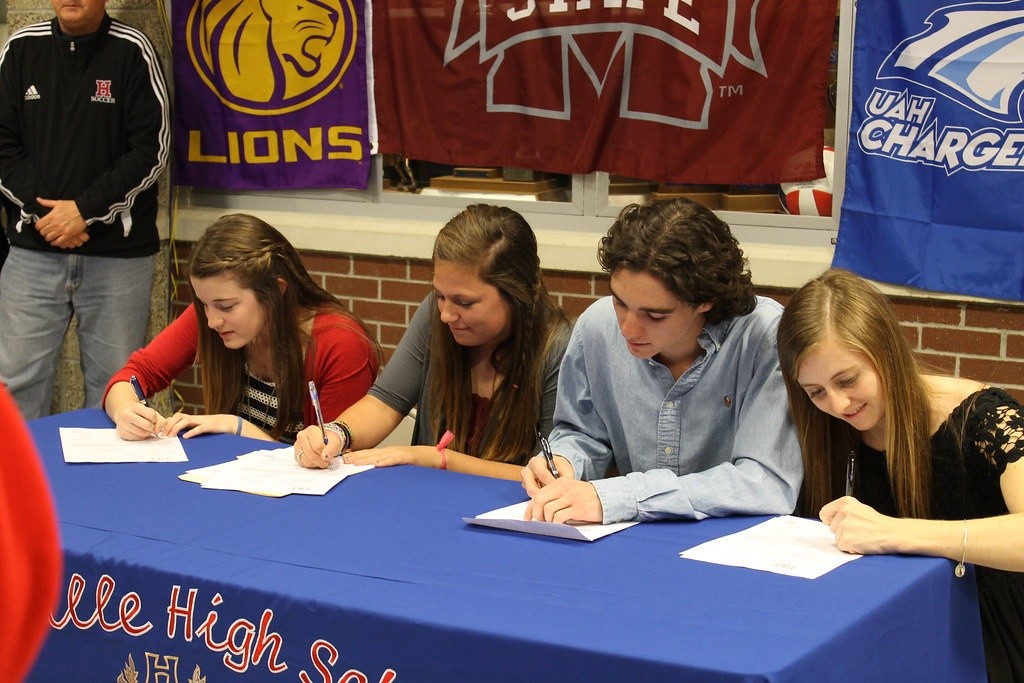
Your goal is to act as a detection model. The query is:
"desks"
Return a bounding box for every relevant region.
[25,408,986,683]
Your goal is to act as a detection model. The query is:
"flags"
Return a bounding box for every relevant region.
[365,0,835,192]
[826,1,1024,303]
[166,0,380,192]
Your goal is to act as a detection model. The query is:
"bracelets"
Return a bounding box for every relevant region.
[322,416,354,458]
[953,518,968,577]
[236,413,243,436]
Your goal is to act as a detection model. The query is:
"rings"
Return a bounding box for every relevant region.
[297,449,303,461]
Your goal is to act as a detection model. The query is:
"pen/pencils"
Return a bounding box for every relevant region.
[845,450,857,497]
[129,374,160,440]
[537,431,559,479]
[308,380,335,471]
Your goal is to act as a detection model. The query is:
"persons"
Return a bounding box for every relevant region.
[776,267,1023,683]
[102,212,381,459]
[519,195,809,526]
[0,0,173,430]
[292,202,573,484]
